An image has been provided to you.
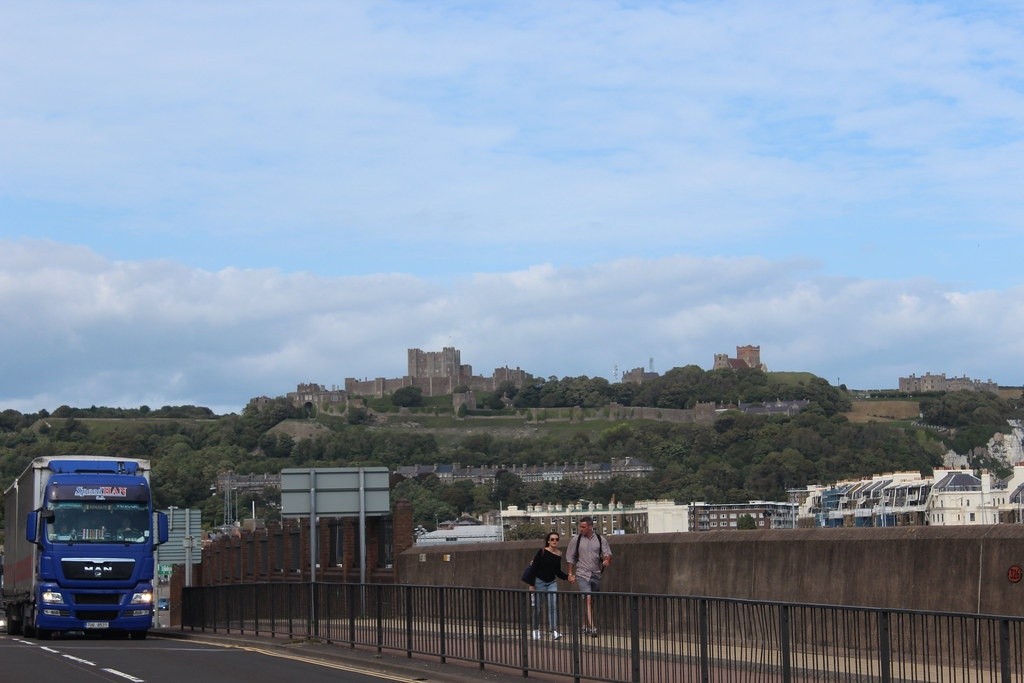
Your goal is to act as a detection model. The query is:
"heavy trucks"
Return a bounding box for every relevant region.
[2,455,169,641]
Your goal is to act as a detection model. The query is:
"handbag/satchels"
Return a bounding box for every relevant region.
[521,547,545,584]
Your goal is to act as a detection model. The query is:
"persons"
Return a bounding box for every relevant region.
[116,514,143,541]
[56,517,77,538]
[530,532,576,639]
[566,516,612,638]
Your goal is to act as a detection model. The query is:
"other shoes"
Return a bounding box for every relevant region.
[552,631,563,640]
[533,630,542,641]
[583,629,590,634]
[591,627,598,637]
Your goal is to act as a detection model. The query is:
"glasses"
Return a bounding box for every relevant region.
[551,538,559,541]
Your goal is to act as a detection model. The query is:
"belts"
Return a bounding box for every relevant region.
[545,581,557,586]
